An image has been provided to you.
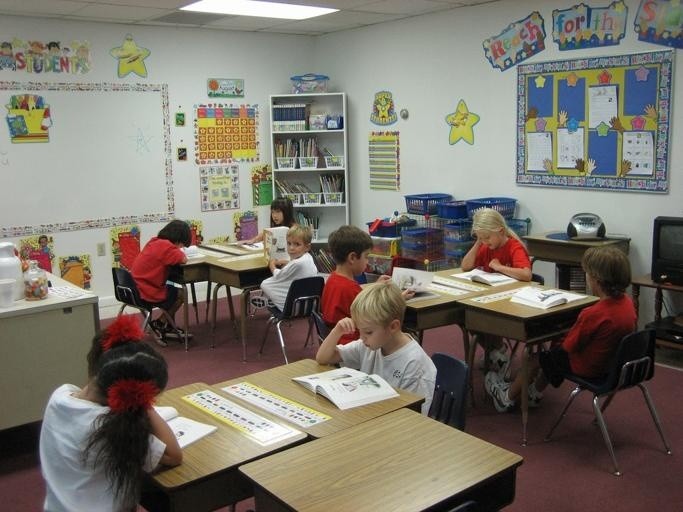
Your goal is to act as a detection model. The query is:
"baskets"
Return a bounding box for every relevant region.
[465,197,518,221]
[405,193,452,215]
[277,155,344,170]
[282,191,343,206]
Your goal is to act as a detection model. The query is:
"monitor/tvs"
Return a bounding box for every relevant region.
[652,216,683,287]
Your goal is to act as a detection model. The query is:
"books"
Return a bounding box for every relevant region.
[180,246,206,259]
[152,403,219,451]
[292,365,399,410]
[451,267,516,288]
[392,267,441,305]
[510,285,588,311]
[263,226,290,263]
[307,248,338,274]
[272,98,345,240]
[230,242,265,251]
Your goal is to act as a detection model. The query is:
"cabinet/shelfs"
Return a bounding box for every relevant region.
[268,92,349,245]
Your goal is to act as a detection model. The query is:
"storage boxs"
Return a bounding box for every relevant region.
[364,202,475,283]
[290,73,330,92]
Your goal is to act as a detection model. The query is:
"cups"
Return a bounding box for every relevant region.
[0,279,16,308]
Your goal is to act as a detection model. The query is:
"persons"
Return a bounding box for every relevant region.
[129,219,194,348]
[314,280,440,417]
[484,246,638,415]
[35,234,50,272]
[236,197,297,246]
[248,226,320,326]
[318,225,416,346]
[460,206,533,379]
[38,314,184,511]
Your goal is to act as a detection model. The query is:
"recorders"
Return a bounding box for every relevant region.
[568,213,606,240]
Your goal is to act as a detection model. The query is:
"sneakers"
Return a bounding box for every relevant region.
[478,342,545,413]
[145,320,194,347]
[249,296,276,310]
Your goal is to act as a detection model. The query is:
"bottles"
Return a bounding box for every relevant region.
[24,260,49,300]
[0,242,23,301]
